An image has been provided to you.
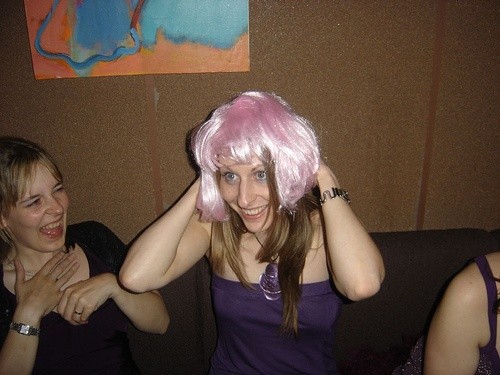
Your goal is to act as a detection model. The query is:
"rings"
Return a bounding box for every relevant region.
[73,310,83,315]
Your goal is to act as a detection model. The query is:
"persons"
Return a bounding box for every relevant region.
[119,91,385,375]
[395,252,500,375]
[0,136,170,375]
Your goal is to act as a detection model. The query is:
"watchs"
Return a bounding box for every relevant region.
[10,321,41,337]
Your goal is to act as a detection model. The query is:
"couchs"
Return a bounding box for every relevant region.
[124,227,500,375]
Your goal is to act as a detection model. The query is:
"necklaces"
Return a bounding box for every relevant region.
[253,232,283,300]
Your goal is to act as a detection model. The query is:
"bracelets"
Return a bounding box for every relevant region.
[318,187,352,206]
